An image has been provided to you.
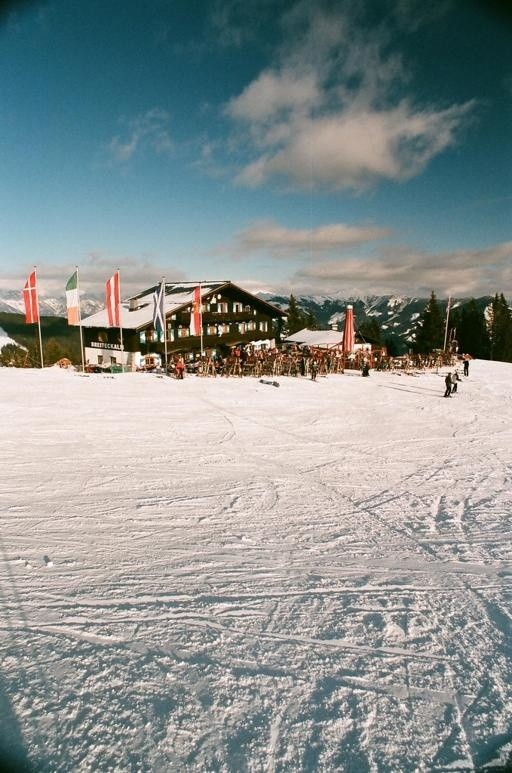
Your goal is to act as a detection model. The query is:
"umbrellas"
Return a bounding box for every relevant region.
[342,304,354,355]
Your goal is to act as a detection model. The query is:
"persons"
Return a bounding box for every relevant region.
[167,342,473,380]
[442,372,455,398]
[450,371,463,394]
